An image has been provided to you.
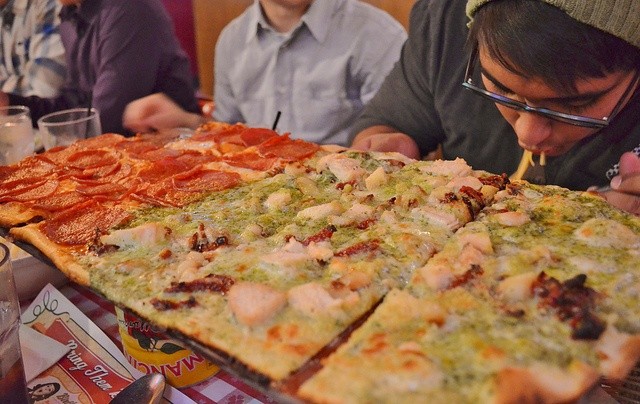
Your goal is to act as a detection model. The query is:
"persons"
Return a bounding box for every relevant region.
[0,0,63,138]
[351,0,640,215]
[0,1,202,131]
[122,0,436,160]
[0,0,68,99]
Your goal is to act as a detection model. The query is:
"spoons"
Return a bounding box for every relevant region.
[109,373,166,404]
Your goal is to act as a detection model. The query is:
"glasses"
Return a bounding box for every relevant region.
[459,41,635,128]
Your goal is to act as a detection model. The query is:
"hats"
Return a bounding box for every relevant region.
[464,1,640,45]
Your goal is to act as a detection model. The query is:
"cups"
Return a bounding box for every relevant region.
[37,108,101,152]
[0,243,31,404]
[0,106,35,167]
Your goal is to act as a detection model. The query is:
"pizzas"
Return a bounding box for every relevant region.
[65,148,508,387]
[0,119,336,273]
[297,178,640,403]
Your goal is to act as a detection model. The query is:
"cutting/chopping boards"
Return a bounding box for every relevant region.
[0,214,384,402]
